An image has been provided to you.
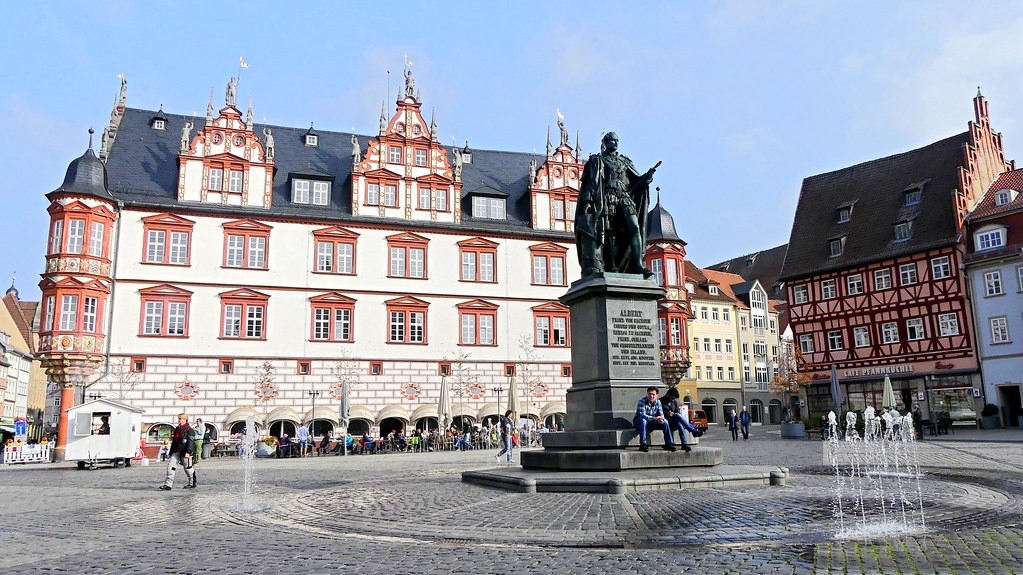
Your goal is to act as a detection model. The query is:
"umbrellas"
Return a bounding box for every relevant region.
[339,376,350,455]
[507,375,520,427]
[831,365,844,425]
[438,373,454,436]
[882,374,897,412]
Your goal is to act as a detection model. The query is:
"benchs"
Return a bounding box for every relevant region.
[805,429,824,441]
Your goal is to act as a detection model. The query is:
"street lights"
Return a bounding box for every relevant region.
[494,387,503,433]
[309,390,319,440]
[924,374,938,436]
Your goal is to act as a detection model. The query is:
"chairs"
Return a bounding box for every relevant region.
[277,432,545,458]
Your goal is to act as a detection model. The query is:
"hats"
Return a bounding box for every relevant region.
[178,413,187,419]
[101,415,108,419]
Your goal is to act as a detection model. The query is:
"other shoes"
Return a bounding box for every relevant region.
[495,454,500,462]
[156,460,160,462]
[159,485,171,490]
[507,460,515,462]
[193,464,198,466]
[681,444,691,451]
[693,431,703,437]
[641,444,649,452]
[184,485,196,489]
[663,443,677,451]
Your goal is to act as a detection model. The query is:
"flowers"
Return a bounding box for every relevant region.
[256,436,280,447]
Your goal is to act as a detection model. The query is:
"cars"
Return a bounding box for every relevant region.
[689,410,708,435]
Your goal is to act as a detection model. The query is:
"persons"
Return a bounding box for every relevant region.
[119,79,127,101]
[236,436,250,458]
[494,410,515,463]
[320,432,332,454]
[181,76,274,157]
[280,433,299,454]
[729,405,751,442]
[528,122,568,186]
[574,132,654,277]
[913,405,923,440]
[94,416,109,434]
[298,424,309,458]
[156,413,211,490]
[346,423,549,455]
[633,386,704,451]
[819,415,829,441]
[31,437,38,444]
[348,70,462,171]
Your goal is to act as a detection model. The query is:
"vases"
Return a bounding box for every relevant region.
[257,441,277,457]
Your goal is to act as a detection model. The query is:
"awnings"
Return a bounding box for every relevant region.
[226,401,567,425]
